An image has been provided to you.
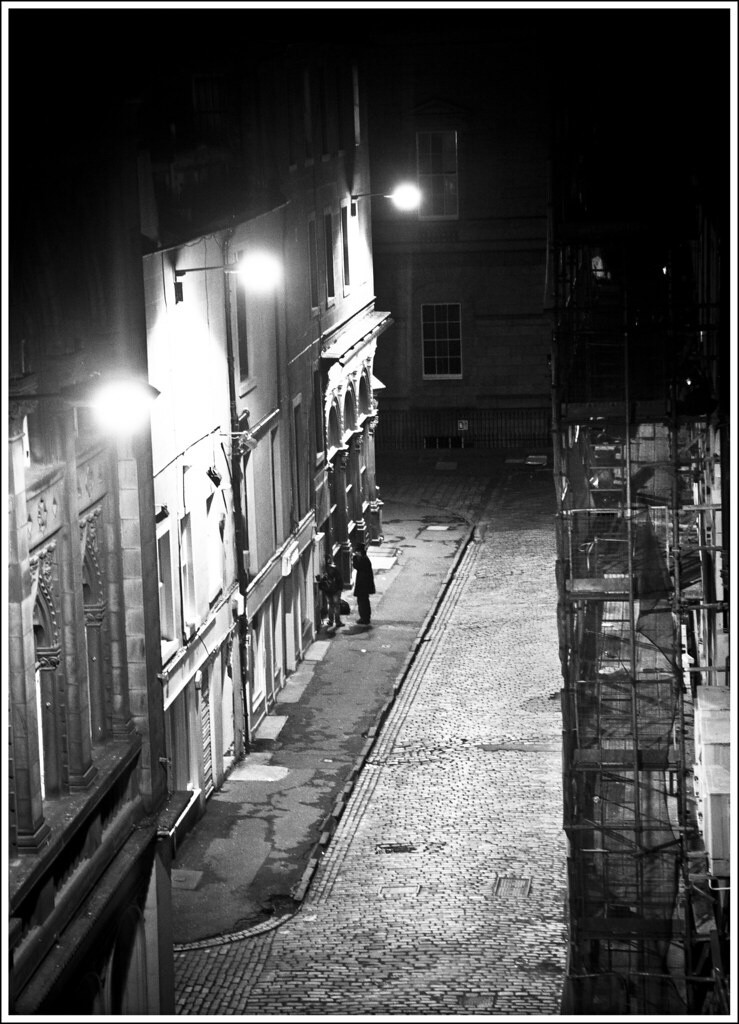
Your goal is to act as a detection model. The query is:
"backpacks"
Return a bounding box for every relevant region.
[318,576,329,593]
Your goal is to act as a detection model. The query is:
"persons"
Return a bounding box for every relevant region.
[351,549,375,624]
[321,554,345,627]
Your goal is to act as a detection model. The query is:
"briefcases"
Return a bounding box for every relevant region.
[340,600,350,615]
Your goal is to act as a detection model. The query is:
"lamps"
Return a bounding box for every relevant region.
[350,185,421,217]
[9,371,163,410]
[174,253,284,304]
[219,430,258,450]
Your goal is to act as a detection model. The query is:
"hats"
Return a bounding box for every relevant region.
[355,543,367,553]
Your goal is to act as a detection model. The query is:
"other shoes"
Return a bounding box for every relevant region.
[357,618,371,624]
[326,619,345,626]
